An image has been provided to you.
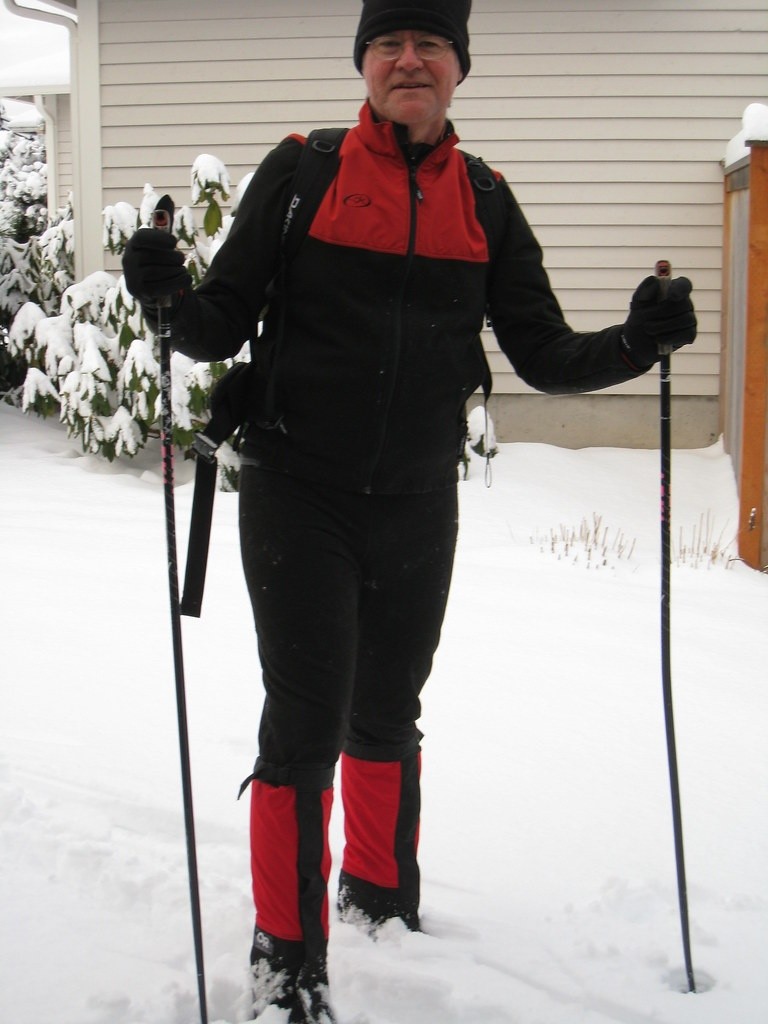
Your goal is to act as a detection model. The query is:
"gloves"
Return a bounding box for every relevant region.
[122,194,193,304]
[626,274,697,359]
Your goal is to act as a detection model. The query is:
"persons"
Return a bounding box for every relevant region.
[122,1,697,1024]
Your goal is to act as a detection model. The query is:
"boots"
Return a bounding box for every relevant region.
[250,756,336,1024]
[339,744,421,933]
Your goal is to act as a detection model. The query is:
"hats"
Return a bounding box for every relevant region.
[353,0,472,87]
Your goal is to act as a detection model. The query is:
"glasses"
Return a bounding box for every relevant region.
[366,34,453,62]
